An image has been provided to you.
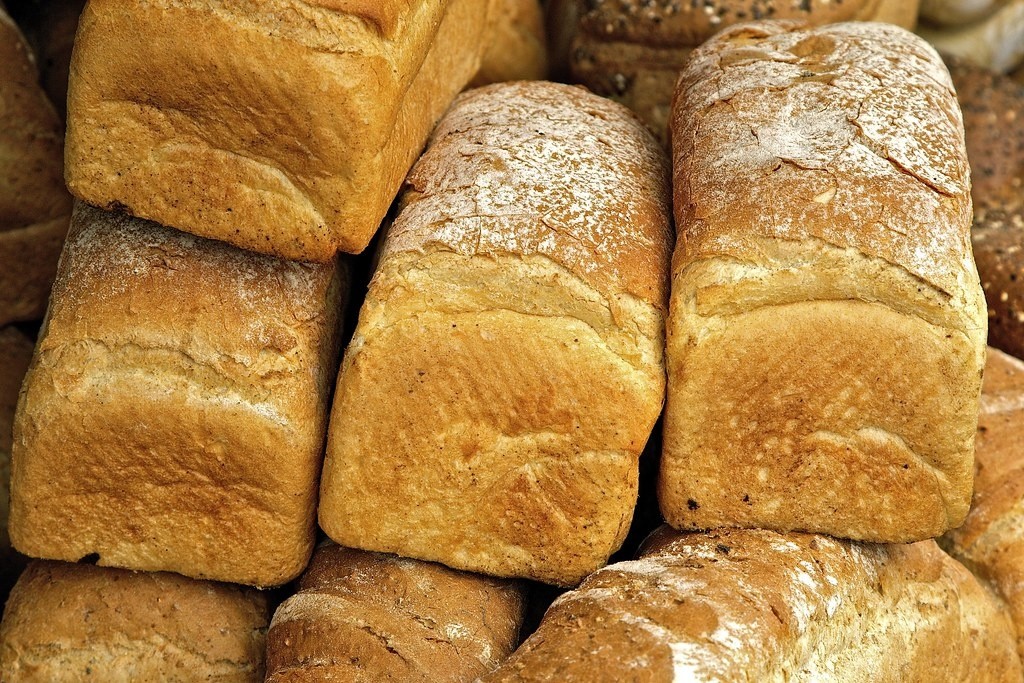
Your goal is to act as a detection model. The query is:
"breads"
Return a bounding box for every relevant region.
[0,0,1024,683]
